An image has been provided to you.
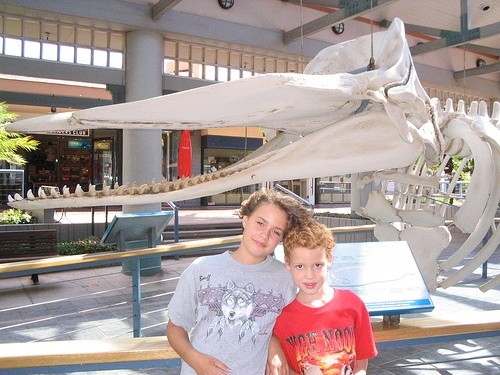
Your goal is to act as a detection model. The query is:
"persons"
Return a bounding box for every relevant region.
[167,188,314,375]
[265,219,378,375]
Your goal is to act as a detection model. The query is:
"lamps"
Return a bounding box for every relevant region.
[477,59,486,67]
[332,22,344,34]
[218,0,234,9]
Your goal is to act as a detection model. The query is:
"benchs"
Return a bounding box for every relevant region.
[0,229,58,285]
